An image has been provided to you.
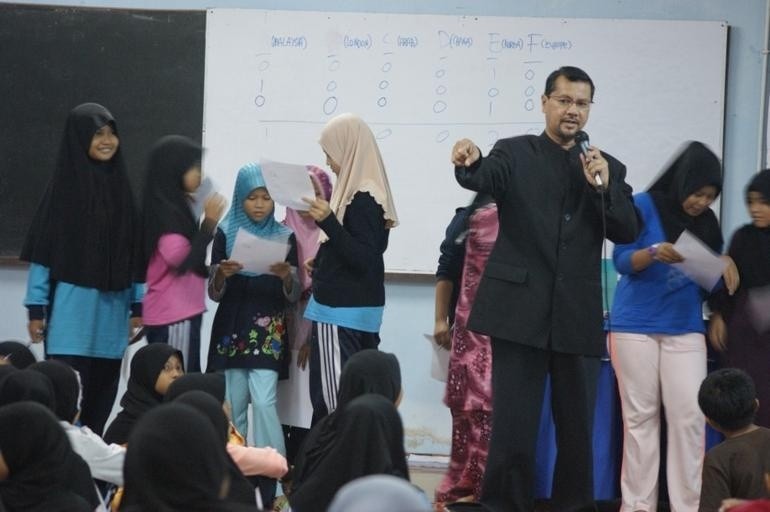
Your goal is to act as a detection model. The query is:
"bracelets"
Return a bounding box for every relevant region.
[647,243,658,262]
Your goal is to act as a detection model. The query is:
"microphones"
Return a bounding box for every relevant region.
[573,129,604,189]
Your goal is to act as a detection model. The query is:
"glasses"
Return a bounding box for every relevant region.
[551,95,595,107]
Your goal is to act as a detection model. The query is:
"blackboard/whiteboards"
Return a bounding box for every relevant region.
[0,3,205,266]
[199,9,729,284]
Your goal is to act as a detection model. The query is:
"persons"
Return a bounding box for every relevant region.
[271,393,411,512]
[0,340,122,511]
[205,161,306,511]
[450,66,645,512]
[697,367,770,511]
[19,102,147,436]
[141,133,228,370]
[706,168,770,429]
[294,349,404,468]
[718,458,770,512]
[433,192,500,511]
[117,401,264,512]
[302,111,401,429]
[174,391,228,450]
[326,472,433,511]
[162,369,290,512]
[279,164,332,466]
[97,342,185,509]
[602,142,741,512]
